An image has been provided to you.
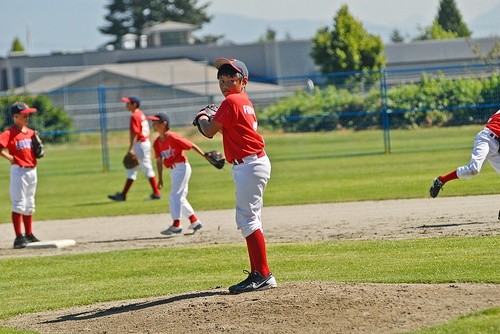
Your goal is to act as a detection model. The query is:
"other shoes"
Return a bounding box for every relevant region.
[14,236,27,248]
[160,225,182,235]
[25,234,41,242]
[107,192,126,201]
[184,220,203,236]
[143,194,161,200]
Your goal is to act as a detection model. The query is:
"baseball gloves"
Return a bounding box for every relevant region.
[193,105,219,124]
[31,129,47,158]
[122,150,139,169]
[204,150,225,169]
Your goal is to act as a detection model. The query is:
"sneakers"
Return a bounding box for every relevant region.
[229,270,277,293]
[430,176,444,198]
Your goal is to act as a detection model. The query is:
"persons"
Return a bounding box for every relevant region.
[0,101,46,248]
[429,110,500,221]
[108,96,163,201]
[146,113,226,236]
[193,57,276,293]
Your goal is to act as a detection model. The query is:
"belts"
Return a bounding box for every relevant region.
[490,133,500,143]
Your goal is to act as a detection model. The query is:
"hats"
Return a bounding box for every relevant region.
[144,112,170,126]
[10,103,37,114]
[122,96,141,106]
[215,57,249,78]
